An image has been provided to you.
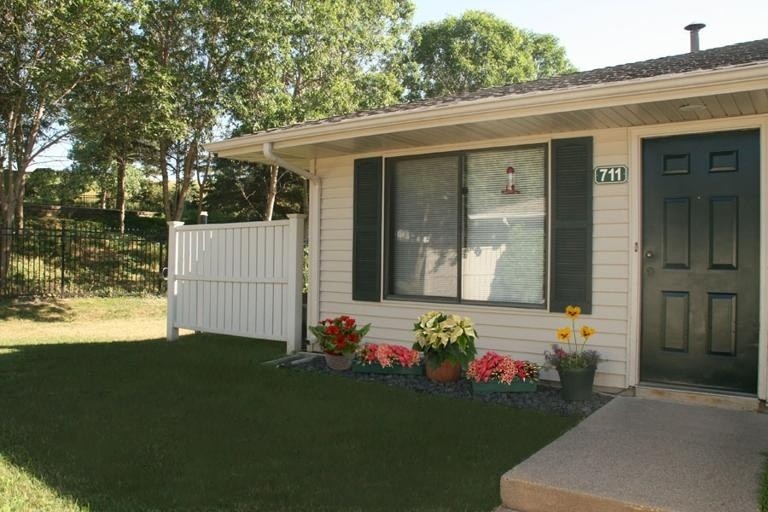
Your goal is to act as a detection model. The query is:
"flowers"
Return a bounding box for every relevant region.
[308,315,373,359]
[544,303,600,367]
[356,341,424,369]
[467,350,542,385]
[412,309,481,368]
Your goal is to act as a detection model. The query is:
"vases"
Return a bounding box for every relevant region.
[351,363,424,376]
[556,367,598,403]
[323,350,357,373]
[468,377,541,397]
[419,356,468,384]
